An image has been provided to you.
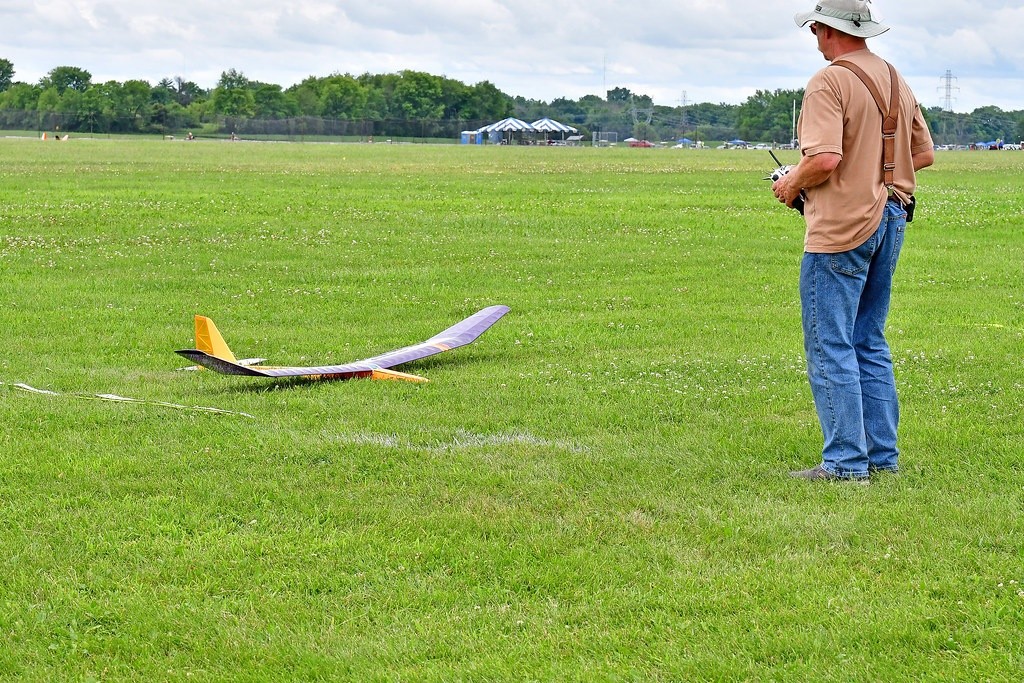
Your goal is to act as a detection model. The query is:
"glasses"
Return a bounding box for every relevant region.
[809,21,826,35]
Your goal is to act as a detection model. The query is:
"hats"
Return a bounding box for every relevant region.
[793,0,890,38]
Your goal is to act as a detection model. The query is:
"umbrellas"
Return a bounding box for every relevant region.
[675,138,692,142]
[477,117,578,146]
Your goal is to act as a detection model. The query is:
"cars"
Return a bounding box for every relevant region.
[747,143,791,150]
[933,144,966,151]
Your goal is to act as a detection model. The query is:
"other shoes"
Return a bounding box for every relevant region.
[792,466,869,485]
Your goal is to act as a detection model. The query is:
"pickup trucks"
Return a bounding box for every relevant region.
[630,139,655,147]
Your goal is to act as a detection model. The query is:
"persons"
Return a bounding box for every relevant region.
[772,0,936,486]
[995,138,1004,150]
